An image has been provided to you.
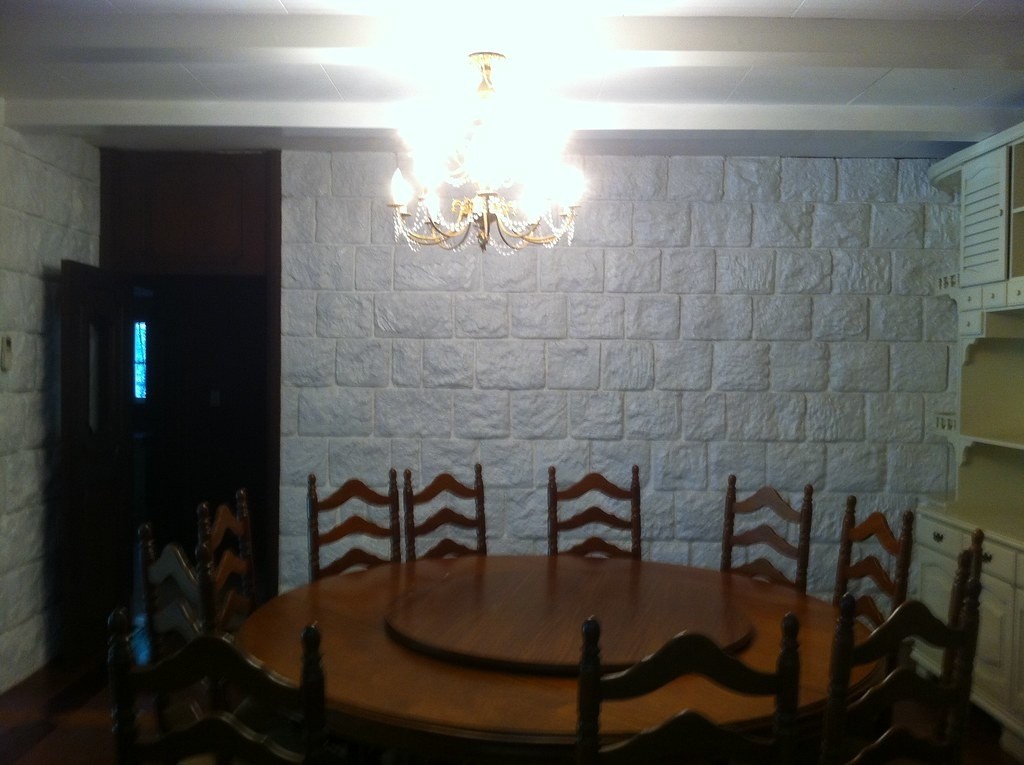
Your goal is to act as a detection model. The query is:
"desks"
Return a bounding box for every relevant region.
[234,553,884,765]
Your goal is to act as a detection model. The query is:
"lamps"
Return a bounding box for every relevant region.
[385,50,584,256]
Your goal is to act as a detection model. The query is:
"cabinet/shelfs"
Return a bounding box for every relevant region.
[910,121,1024,761]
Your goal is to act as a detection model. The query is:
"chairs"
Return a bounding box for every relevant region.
[403,462,486,561]
[307,467,400,582]
[831,494,913,616]
[576,610,802,765]
[545,464,643,560]
[821,529,983,765]
[720,474,814,594]
[136,523,229,740]
[106,608,328,764]
[197,489,262,709]
